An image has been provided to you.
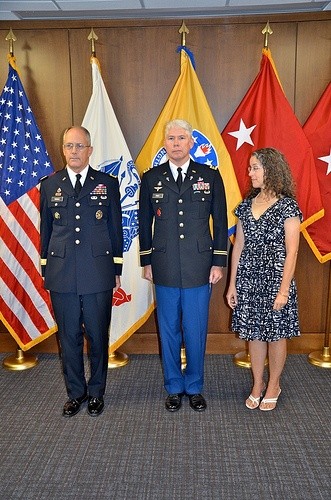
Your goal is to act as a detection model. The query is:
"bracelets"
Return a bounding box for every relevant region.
[279,292,289,298]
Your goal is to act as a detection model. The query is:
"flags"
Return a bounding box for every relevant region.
[134,46,243,247]
[79,57,155,355]
[301,81,331,264]
[220,49,324,232]
[0,54,58,351]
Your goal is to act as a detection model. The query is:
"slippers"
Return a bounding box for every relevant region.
[244,383,266,410]
[259,388,282,412]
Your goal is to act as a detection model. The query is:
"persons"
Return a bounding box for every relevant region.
[40,126,124,418]
[226,148,303,411]
[138,119,228,412]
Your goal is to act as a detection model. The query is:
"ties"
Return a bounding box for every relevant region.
[175,167,183,191]
[74,174,83,198]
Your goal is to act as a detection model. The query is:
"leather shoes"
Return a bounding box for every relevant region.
[63,392,88,417]
[186,390,207,412]
[165,390,185,412]
[86,392,105,417]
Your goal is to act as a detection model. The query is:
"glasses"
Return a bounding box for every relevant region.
[64,143,90,151]
[247,167,260,172]
